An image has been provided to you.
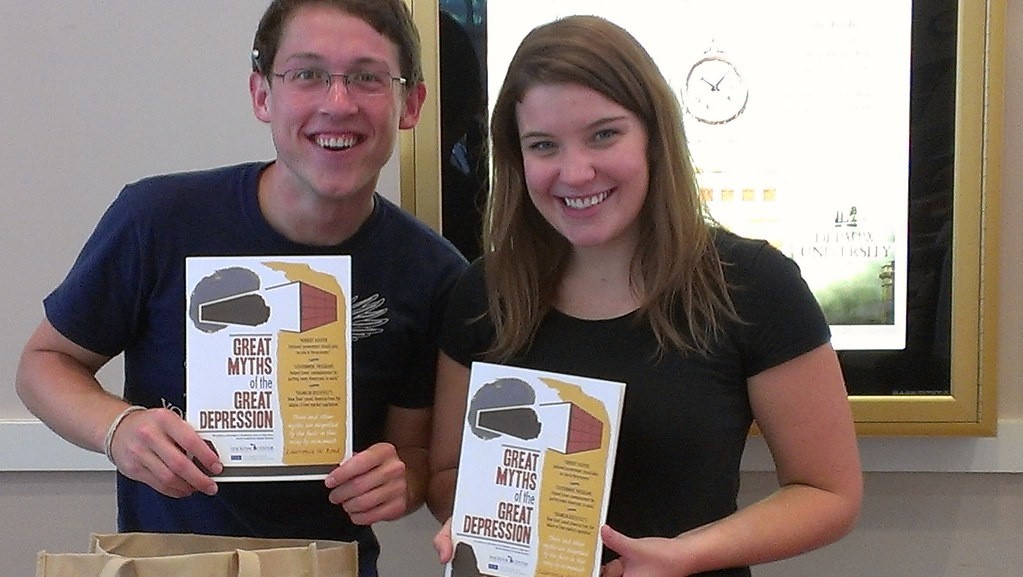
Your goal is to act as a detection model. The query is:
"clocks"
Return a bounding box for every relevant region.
[682,51,756,126]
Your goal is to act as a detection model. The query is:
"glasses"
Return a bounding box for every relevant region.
[262,69,406,96]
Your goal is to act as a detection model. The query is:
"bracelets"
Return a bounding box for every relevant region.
[104,404,148,468]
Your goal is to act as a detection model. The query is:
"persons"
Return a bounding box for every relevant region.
[10,1,470,576]
[427,12,866,576]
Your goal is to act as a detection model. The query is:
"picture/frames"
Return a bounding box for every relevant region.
[386,0,1010,448]
